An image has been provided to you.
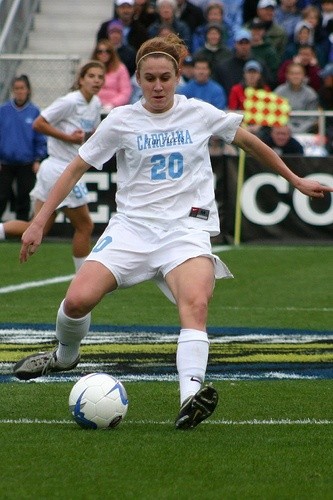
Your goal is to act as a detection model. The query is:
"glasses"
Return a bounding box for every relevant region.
[95,49,112,54]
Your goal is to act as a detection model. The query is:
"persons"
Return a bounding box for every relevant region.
[74,0,333,158]
[0,60,105,273]
[13,34,333,429]
[0,75,48,242]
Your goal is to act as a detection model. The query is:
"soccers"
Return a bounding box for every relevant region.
[68,372,129,430]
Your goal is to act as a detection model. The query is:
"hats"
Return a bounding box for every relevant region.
[258,0,277,9]
[107,20,124,33]
[116,0,135,6]
[234,28,252,42]
[243,60,262,72]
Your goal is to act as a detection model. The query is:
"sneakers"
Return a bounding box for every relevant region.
[174,386,218,430]
[13,349,81,380]
